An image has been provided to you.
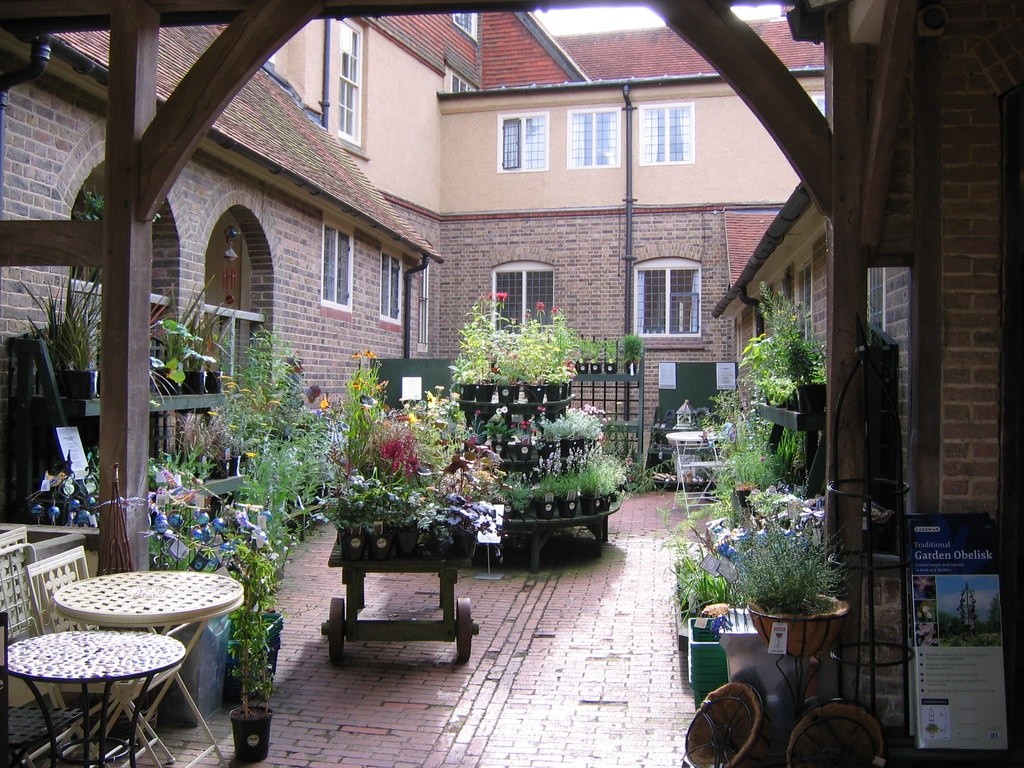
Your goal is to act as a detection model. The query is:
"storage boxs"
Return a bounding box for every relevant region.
[687,616,728,714]
[224,611,283,704]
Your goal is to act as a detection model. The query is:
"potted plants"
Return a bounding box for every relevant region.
[738,277,825,415]
[572,333,644,375]
[724,473,850,656]
[20,265,259,485]
[222,542,282,764]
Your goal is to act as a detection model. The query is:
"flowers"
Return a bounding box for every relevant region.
[450,288,627,512]
[316,349,512,566]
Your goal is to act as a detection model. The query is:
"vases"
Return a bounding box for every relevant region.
[491,440,509,459]
[580,497,602,516]
[534,439,556,460]
[458,383,476,401]
[515,442,532,461]
[422,531,465,560]
[560,437,585,458]
[337,531,366,562]
[533,499,557,519]
[685,682,775,768]
[523,384,550,403]
[555,496,578,518]
[601,494,610,511]
[544,384,564,401]
[366,532,395,562]
[474,384,495,402]
[785,703,883,768]
[498,385,520,403]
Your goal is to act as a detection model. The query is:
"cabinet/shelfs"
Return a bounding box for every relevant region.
[7,323,257,575]
[570,346,715,499]
[452,390,625,574]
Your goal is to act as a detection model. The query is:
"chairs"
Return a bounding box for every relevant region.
[0,545,176,768]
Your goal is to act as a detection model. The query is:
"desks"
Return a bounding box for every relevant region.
[53,571,244,768]
[6,630,187,768]
[664,431,741,519]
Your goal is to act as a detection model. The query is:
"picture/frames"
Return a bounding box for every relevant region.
[393,526,421,558]
